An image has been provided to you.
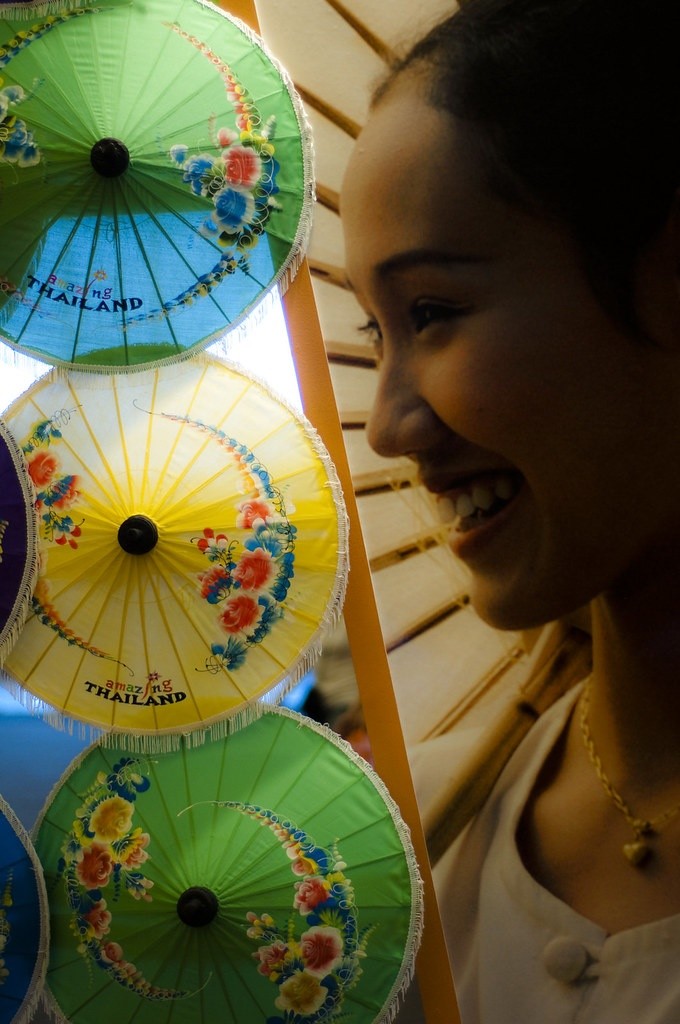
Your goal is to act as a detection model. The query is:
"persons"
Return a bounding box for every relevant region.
[338,0,680,1023]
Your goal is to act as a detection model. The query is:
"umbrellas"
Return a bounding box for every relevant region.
[0,794,50,1022]
[42,701,424,1014]
[0,1,316,376]
[0,347,353,753]
[0,422,39,665]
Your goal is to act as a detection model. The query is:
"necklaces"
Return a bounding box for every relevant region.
[580,675,680,864]
